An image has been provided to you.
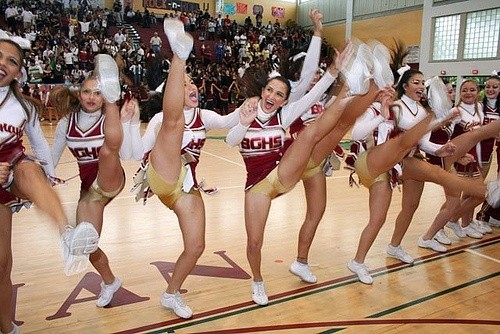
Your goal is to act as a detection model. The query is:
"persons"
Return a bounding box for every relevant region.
[0,0,237,122]
[53,54,135,306]
[126,18,256,319]
[226,9,394,305]
[0,39,99,334]
[347,39,500,284]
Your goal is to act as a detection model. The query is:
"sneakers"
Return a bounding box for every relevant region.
[427,86,450,120]
[95,53,121,104]
[346,259,373,284]
[344,43,374,95]
[59,222,98,278]
[0,322,21,334]
[163,17,195,60]
[159,290,193,318]
[252,278,269,305]
[95,277,122,306]
[288,261,317,283]
[371,45,395,90]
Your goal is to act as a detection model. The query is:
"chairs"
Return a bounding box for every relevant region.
[0,0,340,122]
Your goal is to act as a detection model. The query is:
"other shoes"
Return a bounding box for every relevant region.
[435,228,452,244]
[385,242,414,263]
[418,236,447,252]
[460,224,483,238]
[474,218,493,234]
[470,221,486,234]
[446,221,467,238]
[488,216,500,228]
